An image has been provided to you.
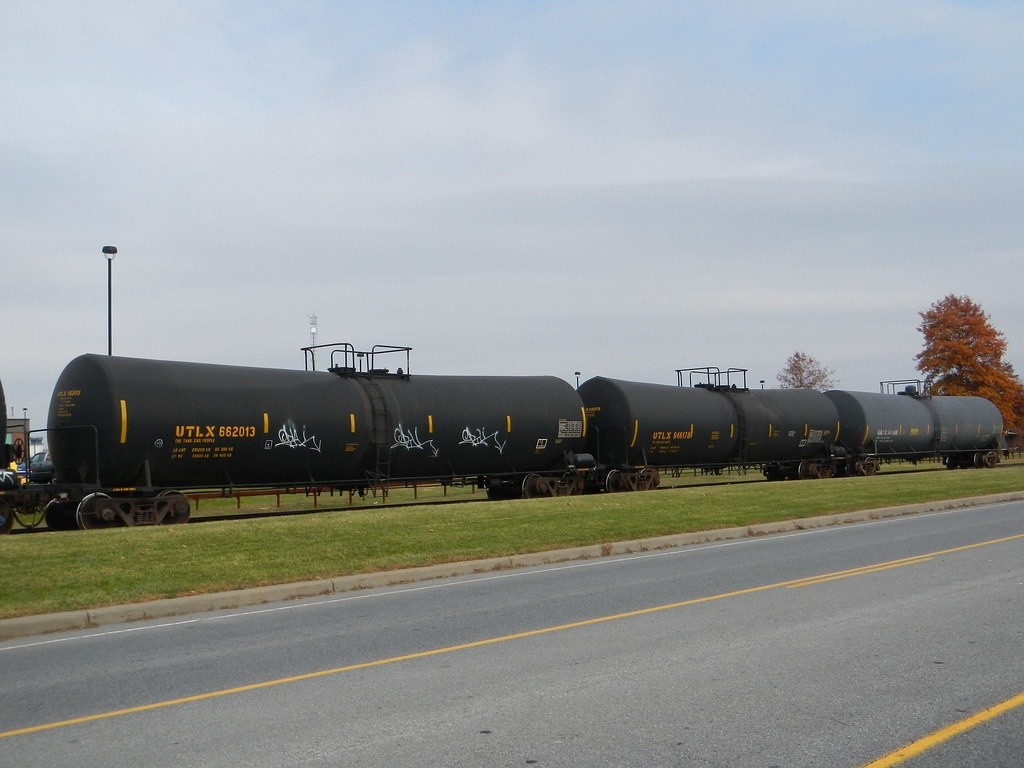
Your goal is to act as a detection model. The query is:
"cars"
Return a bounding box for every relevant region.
[17,449,57,486]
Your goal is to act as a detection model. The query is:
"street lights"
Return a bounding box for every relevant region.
[575,372,581,389]
[22,408,28,420]
[103,246,118,356]
[759,380,765,389]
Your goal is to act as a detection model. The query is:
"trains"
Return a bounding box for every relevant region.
[0,343,1021,535]
